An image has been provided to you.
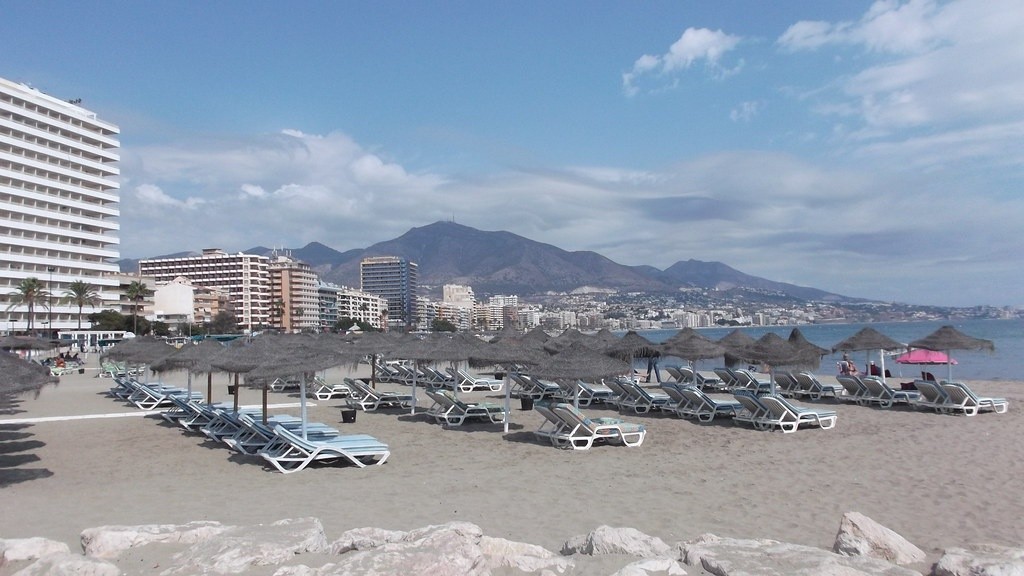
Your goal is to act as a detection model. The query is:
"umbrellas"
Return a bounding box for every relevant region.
[101,326,994,441]
[0,336,61,397]
[896,349,958,380]
[878,342,917,378]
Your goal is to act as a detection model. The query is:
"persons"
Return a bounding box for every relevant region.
[843,351,849,362]
[60,350,78,361]
[646,356,663,383]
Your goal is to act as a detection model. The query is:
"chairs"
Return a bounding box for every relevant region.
[33,354,86,378]
[108,329,1012,476]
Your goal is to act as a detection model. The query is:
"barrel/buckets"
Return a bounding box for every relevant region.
[227,385,237,395]
[78,369,86,374]
[494,371,505,381]
[341,409,357,423]
[519,394,534,410]
